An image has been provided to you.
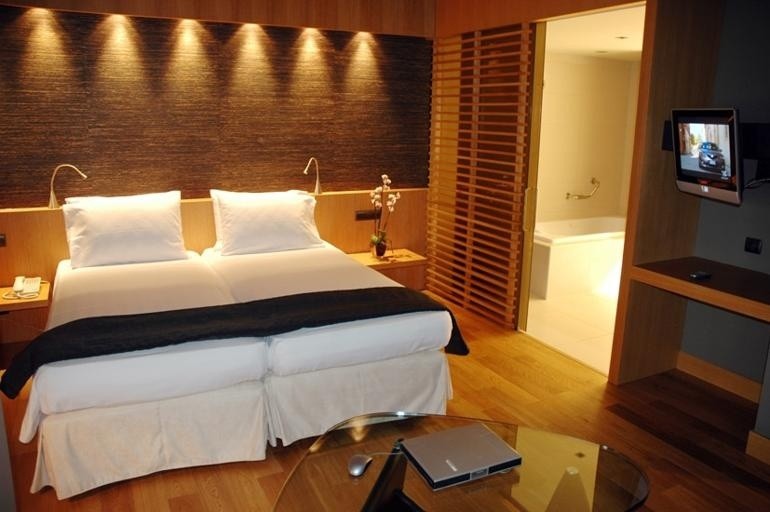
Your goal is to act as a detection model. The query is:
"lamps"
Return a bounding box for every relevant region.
[303,157,321,194]
[49,164,88,209]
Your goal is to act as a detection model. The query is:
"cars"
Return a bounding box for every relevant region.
[698,142,725,173]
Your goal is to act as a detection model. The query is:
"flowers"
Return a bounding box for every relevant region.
[370,174,400,244]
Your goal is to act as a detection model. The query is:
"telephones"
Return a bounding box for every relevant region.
[12,276,41,296]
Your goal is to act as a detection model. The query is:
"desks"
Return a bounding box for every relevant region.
[631,257,770,468]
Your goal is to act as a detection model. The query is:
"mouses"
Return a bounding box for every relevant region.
[347,454,372,476]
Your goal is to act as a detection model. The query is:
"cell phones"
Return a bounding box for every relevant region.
[689,270,710,279]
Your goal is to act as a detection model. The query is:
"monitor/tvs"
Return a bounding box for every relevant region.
[670,107,743,207]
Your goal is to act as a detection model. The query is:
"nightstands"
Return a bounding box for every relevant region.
[0,282,53,370]
[348,249,427,290]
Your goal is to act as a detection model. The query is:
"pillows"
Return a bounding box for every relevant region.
[210,189,325,256]
[63,191,189,268]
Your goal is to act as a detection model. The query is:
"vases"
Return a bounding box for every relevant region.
[370,240,386,258]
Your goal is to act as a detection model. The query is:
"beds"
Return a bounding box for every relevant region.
[19,252,268,501]
[201,239,454,447]
